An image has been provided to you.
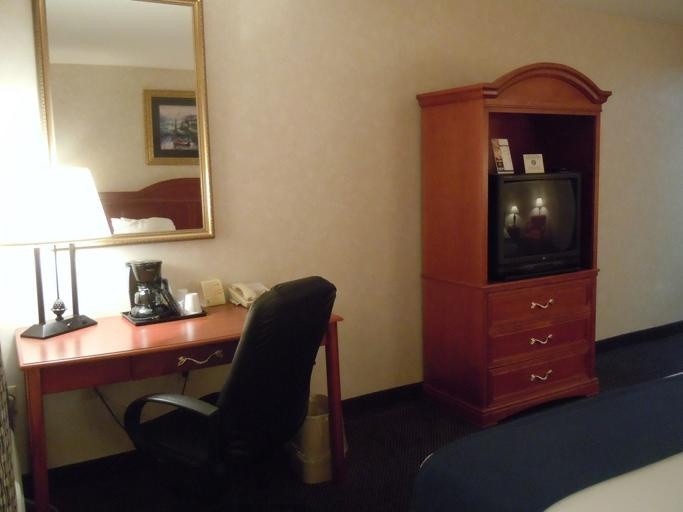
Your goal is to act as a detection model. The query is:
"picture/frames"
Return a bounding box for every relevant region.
[143,90,200,165]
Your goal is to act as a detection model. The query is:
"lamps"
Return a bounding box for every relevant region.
[11,166,114,339]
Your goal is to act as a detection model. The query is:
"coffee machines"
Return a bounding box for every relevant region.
[129,260,162,318]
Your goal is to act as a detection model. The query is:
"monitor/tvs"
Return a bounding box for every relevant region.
[487,173,583,283]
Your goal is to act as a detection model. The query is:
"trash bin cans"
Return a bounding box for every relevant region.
[299,394,333,484]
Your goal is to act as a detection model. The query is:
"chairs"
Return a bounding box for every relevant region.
[124,276,337,511]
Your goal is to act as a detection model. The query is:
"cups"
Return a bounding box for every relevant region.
[184,292,202,315]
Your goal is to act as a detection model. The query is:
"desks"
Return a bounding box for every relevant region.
[14,302,348,512]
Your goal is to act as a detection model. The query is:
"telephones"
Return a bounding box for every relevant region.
[228,281,270,308]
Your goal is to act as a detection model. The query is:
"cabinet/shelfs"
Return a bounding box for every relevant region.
[416,63,615,421]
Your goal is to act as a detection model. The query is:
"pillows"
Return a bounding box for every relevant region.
[110,216,177,235]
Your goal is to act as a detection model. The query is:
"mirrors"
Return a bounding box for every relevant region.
[33,0,217,250]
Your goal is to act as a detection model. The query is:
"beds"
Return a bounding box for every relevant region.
[413,368,681,511]
